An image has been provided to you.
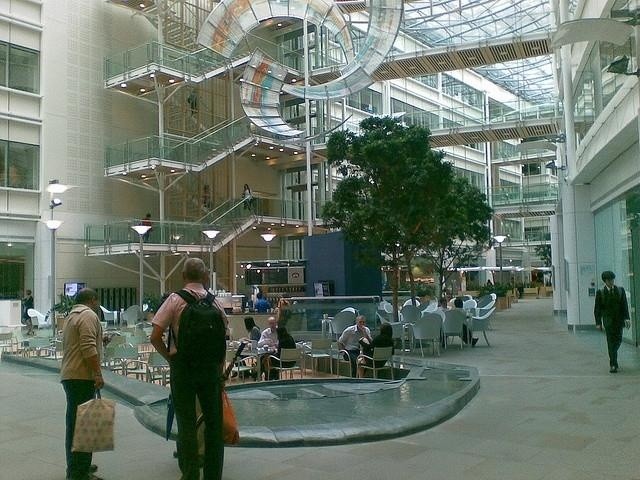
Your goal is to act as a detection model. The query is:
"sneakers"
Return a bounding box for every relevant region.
[66,471,103,480]
[66,464,97,473]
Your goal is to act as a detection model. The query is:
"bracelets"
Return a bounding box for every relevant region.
[95,372,102,376]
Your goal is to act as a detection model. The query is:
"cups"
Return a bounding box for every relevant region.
[271,287,300,293]
[120,308,125,312]
[229,340,269,351]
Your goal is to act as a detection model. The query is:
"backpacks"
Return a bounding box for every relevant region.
[174,289,227,366]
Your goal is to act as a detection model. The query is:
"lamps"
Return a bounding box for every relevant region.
[607,54,640,78]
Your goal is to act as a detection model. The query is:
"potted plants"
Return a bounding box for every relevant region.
[479,282,541,312]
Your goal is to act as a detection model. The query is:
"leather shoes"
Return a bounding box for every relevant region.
[610,362,618,372]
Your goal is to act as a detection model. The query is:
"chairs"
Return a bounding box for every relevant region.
[0,324,64,361]
[119,305,142,328]
[324,307,360,341]
[327,340,404,381]
[99,321,171,386]
[226,339,313,382]
[99,305,118,327]
[378,291,496,357]
[27,308,47,329]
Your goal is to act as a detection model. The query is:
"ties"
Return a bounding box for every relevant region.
[610,288,613,297]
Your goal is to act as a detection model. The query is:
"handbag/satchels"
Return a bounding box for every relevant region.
[70,388,116,453]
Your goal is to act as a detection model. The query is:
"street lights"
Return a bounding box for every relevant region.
[42,176,69,339]
[493,234,508,286]
[130,224,152,322]
[201,229,223,291]
[258,233,278,260]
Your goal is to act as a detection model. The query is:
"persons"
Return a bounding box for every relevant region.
[139,213,153,243]
[487,276,544,299]
[243,184,251,210]
[356,323,394,378]
[434,298,449,348]
[454,299,479,346]
[337,315,373,378]
[231,293,296,382]
[60,288,111,480]
[594,271,631,373]
[22,290,34,335]
[150,258,229,479]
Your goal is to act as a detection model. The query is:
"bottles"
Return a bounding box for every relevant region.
[208,288,232,297]
[224,301,279,314]
